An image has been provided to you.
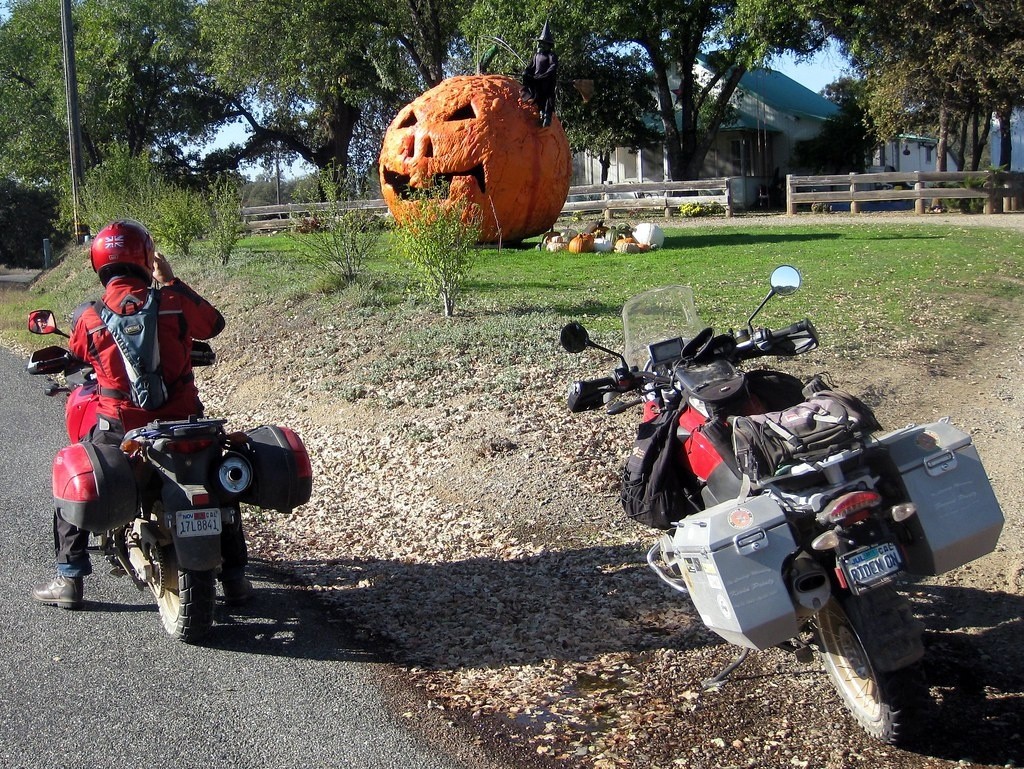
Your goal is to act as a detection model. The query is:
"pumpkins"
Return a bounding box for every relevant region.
[543,219,665,255]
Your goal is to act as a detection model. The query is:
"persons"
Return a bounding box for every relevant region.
[32,220,256,609]
[29,311,55,334]
[521,20,557,128]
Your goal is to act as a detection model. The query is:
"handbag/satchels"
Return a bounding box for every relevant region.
[729,389,880,482]
[621,399,701,530]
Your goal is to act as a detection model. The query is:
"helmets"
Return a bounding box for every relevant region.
[90,222,154,286]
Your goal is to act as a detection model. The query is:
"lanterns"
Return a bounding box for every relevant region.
[379,77,572,242]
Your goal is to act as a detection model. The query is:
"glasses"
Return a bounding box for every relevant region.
[37,319,47,323]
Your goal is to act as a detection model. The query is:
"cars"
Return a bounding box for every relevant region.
[867,164,914,202]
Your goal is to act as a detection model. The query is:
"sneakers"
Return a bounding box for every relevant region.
[32,576,83,609]
[223,579,252,605]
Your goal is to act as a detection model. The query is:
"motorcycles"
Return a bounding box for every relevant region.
[26,308,315,646]
[559,263,1006,746]
